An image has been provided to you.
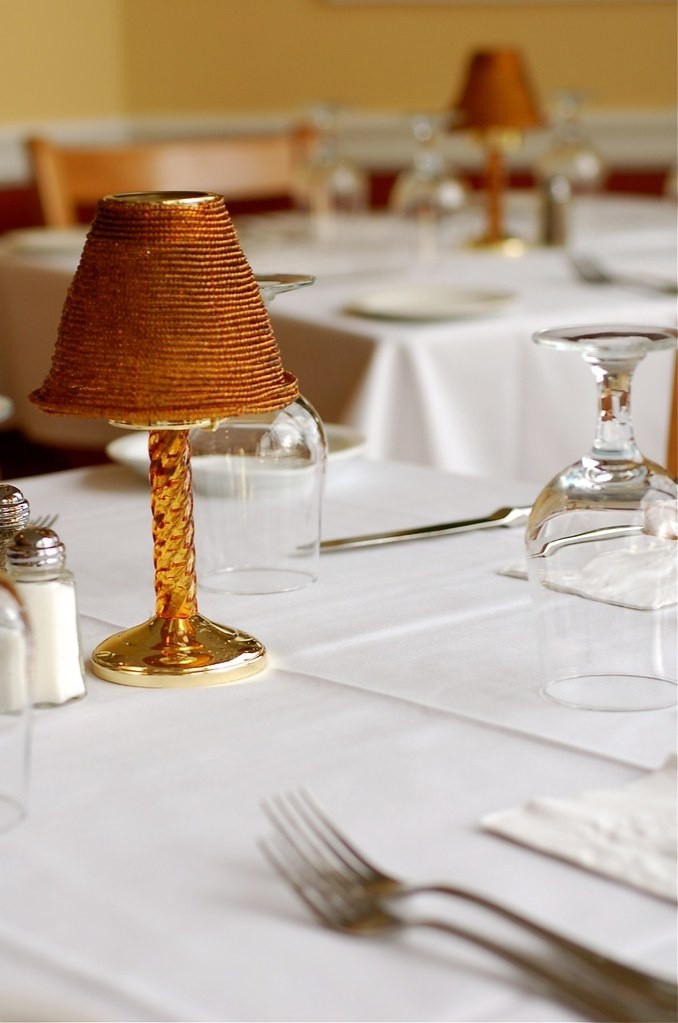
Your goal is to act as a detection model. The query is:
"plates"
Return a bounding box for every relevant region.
[344,285,526,324]
[104,423,365,482]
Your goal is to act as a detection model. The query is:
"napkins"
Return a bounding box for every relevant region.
[498,530,678,610]
[482,754,677,904]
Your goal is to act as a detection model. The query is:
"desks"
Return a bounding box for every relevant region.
[1,454,678,1023]
[0,200,677,483]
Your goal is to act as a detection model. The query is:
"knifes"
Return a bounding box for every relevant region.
[297,504,532,553]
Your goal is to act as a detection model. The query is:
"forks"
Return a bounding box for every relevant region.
[30,513,60,528]
[259,786,678,1023]
[258,837,648,1022]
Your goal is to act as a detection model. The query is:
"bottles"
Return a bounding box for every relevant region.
[0,483,31,586]
[7,526,87,707]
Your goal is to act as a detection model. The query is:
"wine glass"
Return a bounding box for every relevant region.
[526,322,677,712]
[186,273,328,593]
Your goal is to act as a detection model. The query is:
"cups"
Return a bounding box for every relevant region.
[0,576,35,832]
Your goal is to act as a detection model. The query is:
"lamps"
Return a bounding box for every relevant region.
[25,192,301,691]
[446,42,543,255]
